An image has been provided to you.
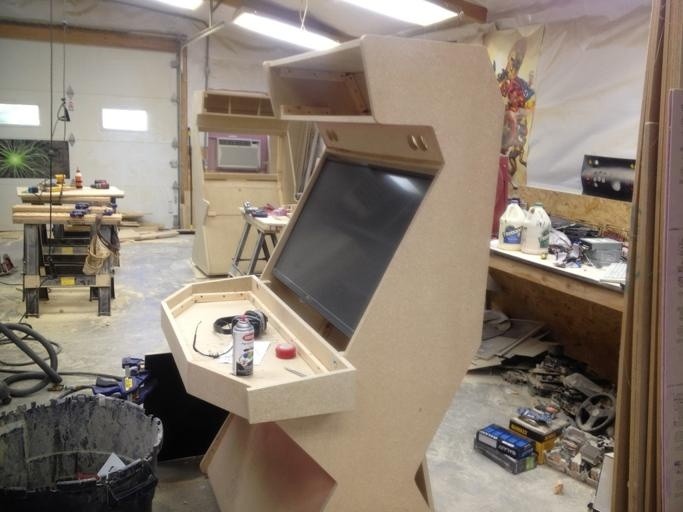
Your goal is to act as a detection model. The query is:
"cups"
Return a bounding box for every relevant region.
[54,175,68,183]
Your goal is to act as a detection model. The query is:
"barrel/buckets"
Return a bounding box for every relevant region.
[520,202,552,255]
[0,392,165,512]
[497,198,527,251]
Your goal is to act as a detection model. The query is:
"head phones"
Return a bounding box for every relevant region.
[213,310,268,334]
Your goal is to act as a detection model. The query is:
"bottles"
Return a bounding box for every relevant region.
[74,167,83,186]
[232,314,253,377]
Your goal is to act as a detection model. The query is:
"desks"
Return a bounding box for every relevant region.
[16,183,124,302]
[226,207,293,279]
[489,185,635,387]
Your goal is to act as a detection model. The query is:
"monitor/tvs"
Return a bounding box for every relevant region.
[271,154,434,340]
